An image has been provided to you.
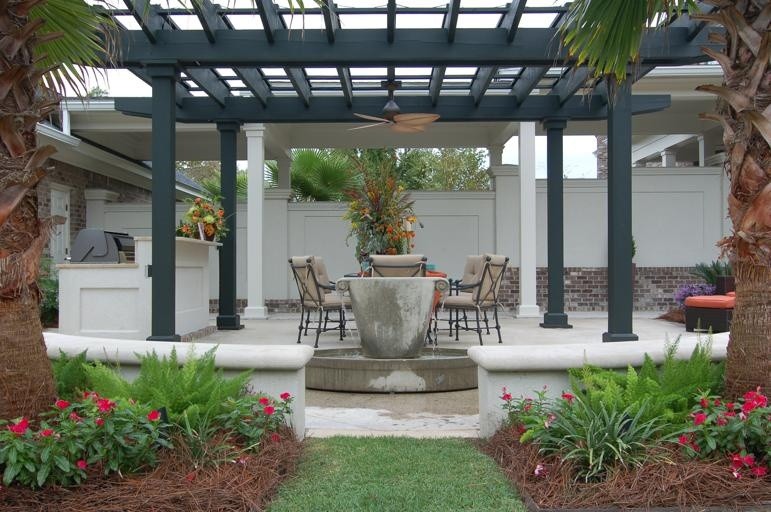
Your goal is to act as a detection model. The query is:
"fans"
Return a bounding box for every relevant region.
[347,95,440,135]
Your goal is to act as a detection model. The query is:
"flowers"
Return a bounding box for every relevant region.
[675,284,716,308]
[337,156,424,278]
[177,196,238,242]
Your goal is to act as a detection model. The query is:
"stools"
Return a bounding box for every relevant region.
[683,291,736,333]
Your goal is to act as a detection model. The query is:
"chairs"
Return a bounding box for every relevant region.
[288,253,510,349]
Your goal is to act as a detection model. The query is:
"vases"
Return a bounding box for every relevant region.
[202,223,217,241]
